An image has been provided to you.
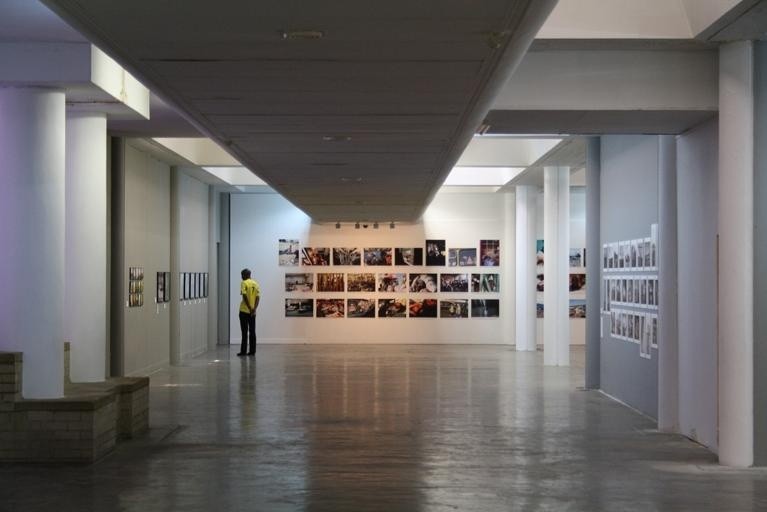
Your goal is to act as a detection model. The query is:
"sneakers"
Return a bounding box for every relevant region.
[236,350,256,356]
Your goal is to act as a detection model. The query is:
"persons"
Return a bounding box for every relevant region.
[237,269,260,356]
